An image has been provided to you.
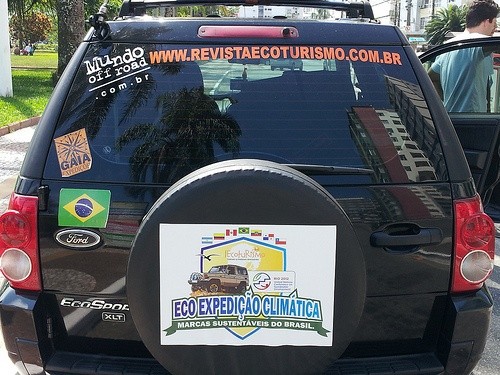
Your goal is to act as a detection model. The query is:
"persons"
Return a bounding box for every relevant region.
[427,0,499,113]
[14,43,36,56]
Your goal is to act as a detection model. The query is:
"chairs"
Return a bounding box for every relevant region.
[161,61,220,131]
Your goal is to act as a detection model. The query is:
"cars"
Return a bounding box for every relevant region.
[270,56,304,72]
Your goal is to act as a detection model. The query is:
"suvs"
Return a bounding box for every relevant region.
[2,2,500,375]
